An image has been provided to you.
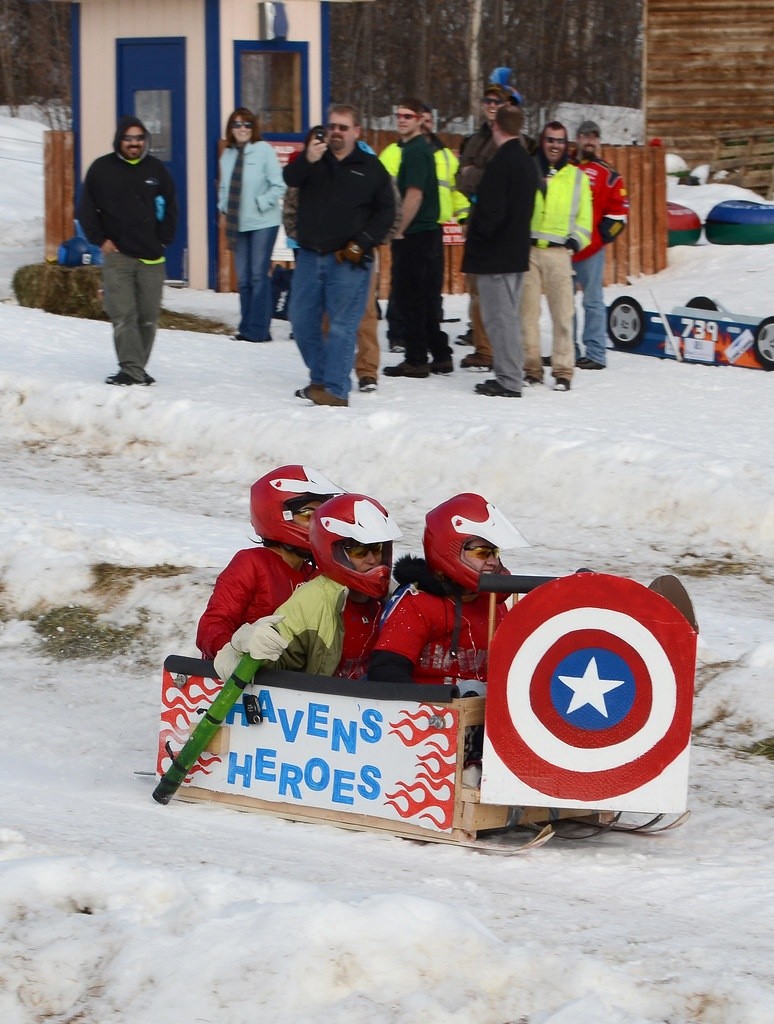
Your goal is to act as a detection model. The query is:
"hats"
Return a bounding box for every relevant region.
[577,121,601,136]
[484,83,510,100]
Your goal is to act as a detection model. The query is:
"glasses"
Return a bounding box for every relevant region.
[292,507,316,522]
[395,113,421,120]
[344,543,383,559]
[543,136,565,144]
[481,97,506,106]
[122,135,145,141]
[328,123,356,132]
[229,121,255,129]
[463,545,500,560]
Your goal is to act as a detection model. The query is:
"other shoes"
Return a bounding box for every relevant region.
[460,352,494,372]
[522,375,570,392]
[454,329,475,346]
[106,371,158,387]
[294,377,377,407]
[389,340,405,353]
[383,359,453,378]
[542,353,606,370]
[473,380,521,397]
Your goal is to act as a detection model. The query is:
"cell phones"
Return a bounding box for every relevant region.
[316,128,326,143]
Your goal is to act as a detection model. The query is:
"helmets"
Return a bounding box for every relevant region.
[309,494,403,600]
[250,465,341,554]
[422,493,533,604]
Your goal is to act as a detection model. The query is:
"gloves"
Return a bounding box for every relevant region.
[214,615,289,684]
[342,241,366,263]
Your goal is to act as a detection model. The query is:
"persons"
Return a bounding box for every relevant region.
[195,463,351,658]
[213,493,404,680]
[365,493,534,698]
[80,116,179,387]
[221,68,629,407]
